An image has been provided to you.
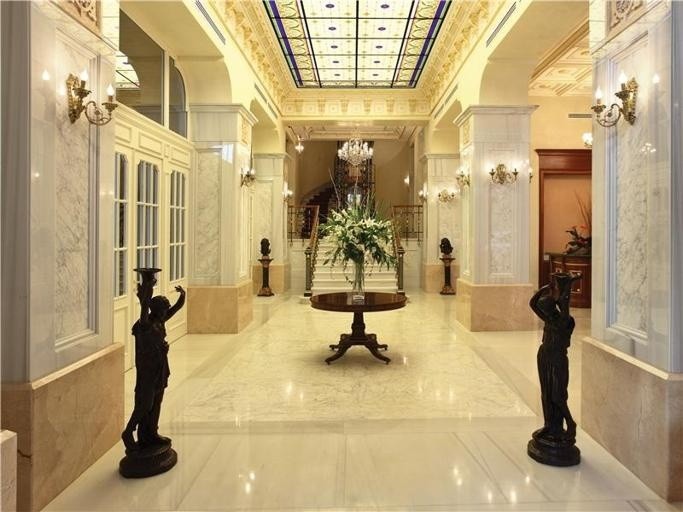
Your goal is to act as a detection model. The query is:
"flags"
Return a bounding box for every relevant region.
[353,261,365,297]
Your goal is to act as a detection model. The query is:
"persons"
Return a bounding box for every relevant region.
[118,273,186,451]
[525,268,579,448]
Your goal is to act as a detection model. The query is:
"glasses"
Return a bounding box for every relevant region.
[337,123,374,165]
[65,68,117,126]
[590,76,637,127]
[294,126,304,153]
[529,167,533,183]
[455,167,471,188]
[582,133,593,148]
[283,189,294,202]
[435,187,457,203]
[417,188,427,200]
[489,164,518,185]
[240,166,256,188]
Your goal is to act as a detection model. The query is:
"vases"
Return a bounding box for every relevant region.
[314,190,408,285]
[565,190,591,254]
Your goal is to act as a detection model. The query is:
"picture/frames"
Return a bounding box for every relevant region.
[310,291,406,365]
